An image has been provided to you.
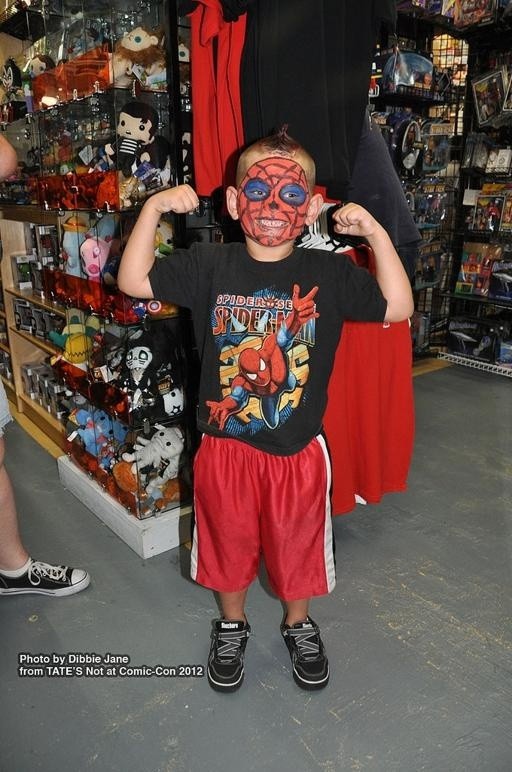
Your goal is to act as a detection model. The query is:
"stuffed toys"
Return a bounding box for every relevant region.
[4,24,183,519]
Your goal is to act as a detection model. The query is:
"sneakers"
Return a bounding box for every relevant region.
[207,614,251,693]
[1,557,91,596]
[281,612,329,691]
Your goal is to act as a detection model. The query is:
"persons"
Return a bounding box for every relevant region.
[116,135,416,695]
[347,109,424,290]
[3,24,183,519]
[0,129,91,596]
